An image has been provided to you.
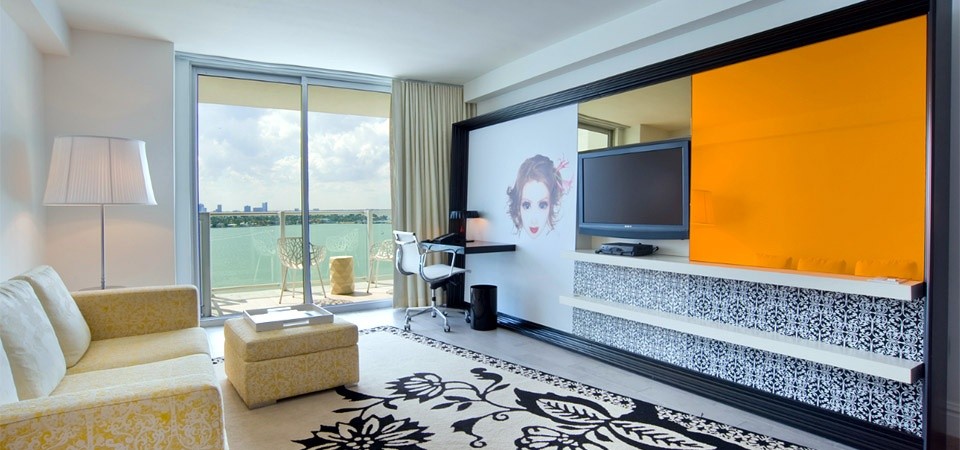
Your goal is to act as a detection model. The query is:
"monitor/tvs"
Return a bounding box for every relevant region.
[578,139,690,239]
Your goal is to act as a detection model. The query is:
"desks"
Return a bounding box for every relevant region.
[419,239,516,315]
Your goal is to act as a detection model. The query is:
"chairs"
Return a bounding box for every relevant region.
[275,236,326,305]
[392,229,471,333]
[366,238,396,294]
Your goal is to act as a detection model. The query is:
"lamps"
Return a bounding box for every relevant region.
[42,134,158,291]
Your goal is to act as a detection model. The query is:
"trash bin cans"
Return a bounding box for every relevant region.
[470,285,497,330]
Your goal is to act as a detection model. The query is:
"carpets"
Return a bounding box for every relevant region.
[210,324,818,450]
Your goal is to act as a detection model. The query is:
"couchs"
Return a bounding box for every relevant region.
[224,316,360,411]
[1,263,228,450]
